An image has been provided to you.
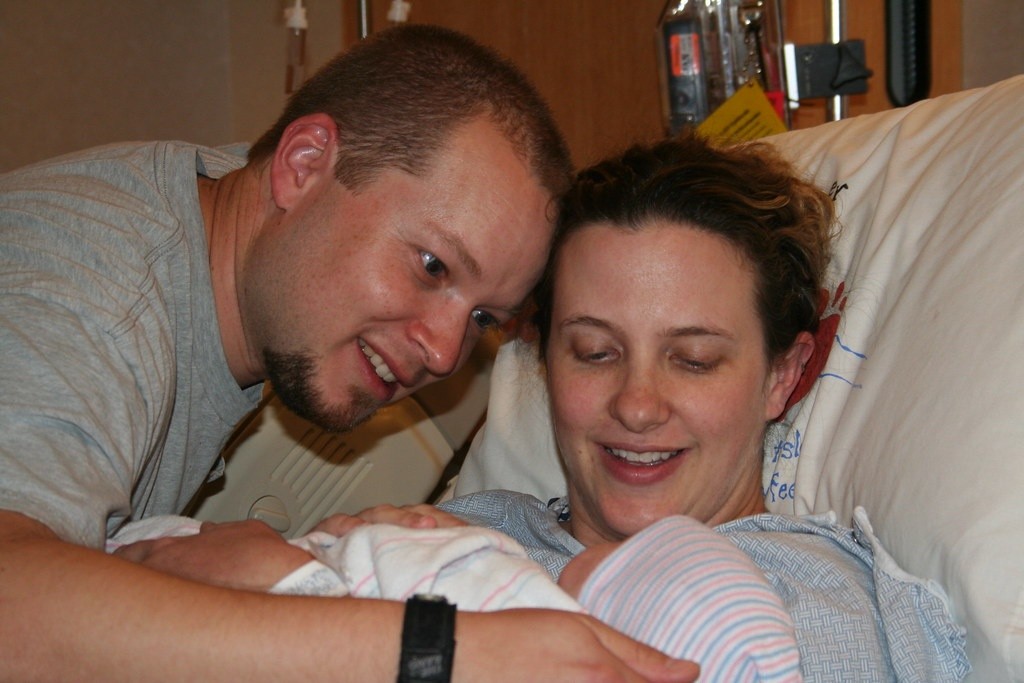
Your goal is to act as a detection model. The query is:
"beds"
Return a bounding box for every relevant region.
[183,77,1024,683]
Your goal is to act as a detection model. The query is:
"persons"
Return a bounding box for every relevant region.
[103,513,805,682]
[0,20,704,683]
[110,133,972,683]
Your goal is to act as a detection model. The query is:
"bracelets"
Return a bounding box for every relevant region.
[394,594,457,683]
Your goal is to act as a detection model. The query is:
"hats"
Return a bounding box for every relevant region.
[577,514,804,683]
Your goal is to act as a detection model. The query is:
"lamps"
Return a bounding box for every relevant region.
[285,1,307,35]
